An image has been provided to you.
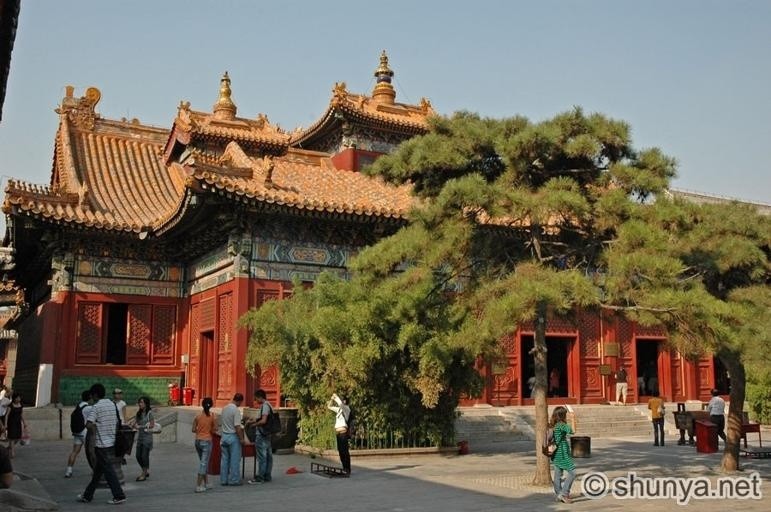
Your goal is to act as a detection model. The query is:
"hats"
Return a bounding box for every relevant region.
[112,388,123,394]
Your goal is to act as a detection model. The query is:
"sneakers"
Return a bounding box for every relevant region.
[557,494,571,503]
[66,466,271,504]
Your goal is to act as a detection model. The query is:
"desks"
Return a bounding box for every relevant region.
[242,443,256,480]
[741,424,761,449]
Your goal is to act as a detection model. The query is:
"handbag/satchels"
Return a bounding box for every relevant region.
[20,431,32,446]
[144,422,162,433]
[116,425,132,457]
[657,405,665,413]
[258,413,281,435]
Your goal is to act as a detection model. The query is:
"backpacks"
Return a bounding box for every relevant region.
[541,429,558,457]
[70,402,88,434]
[342,410,354,436]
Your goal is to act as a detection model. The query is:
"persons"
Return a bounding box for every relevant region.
[707,389,726,443]
[64,382,155,505]
[615,364,628,406]
[548,406,577,503]
[527,365,560,399]
[327,393,351,476]
[637,361,659,397]
[648,391,664,446]
[191,397,217,493]
[219,393,246,486]
[249,389,273,484]
[1,383,27,489]
